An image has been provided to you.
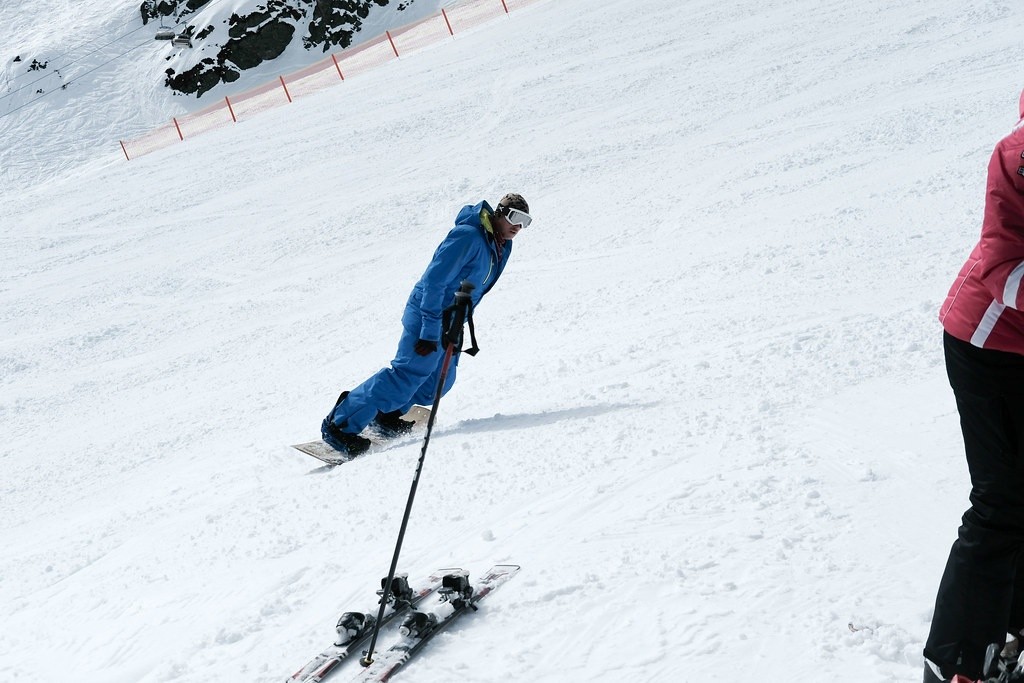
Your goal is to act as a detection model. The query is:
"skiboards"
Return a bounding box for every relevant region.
[282,564,519,683]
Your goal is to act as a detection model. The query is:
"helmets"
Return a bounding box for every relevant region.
[500,193,529,214]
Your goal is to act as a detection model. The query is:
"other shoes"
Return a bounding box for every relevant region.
[370,413,415,435]
[322,418,371,453]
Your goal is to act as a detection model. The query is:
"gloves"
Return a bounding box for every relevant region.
[414,340,438,356]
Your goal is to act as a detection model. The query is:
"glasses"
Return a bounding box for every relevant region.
[497,203,532,229]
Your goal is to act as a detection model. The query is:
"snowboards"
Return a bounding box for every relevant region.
[289,405,437,467]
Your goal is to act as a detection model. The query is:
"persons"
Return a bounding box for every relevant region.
[321,193,529,453]
[922,90,1024,683]
